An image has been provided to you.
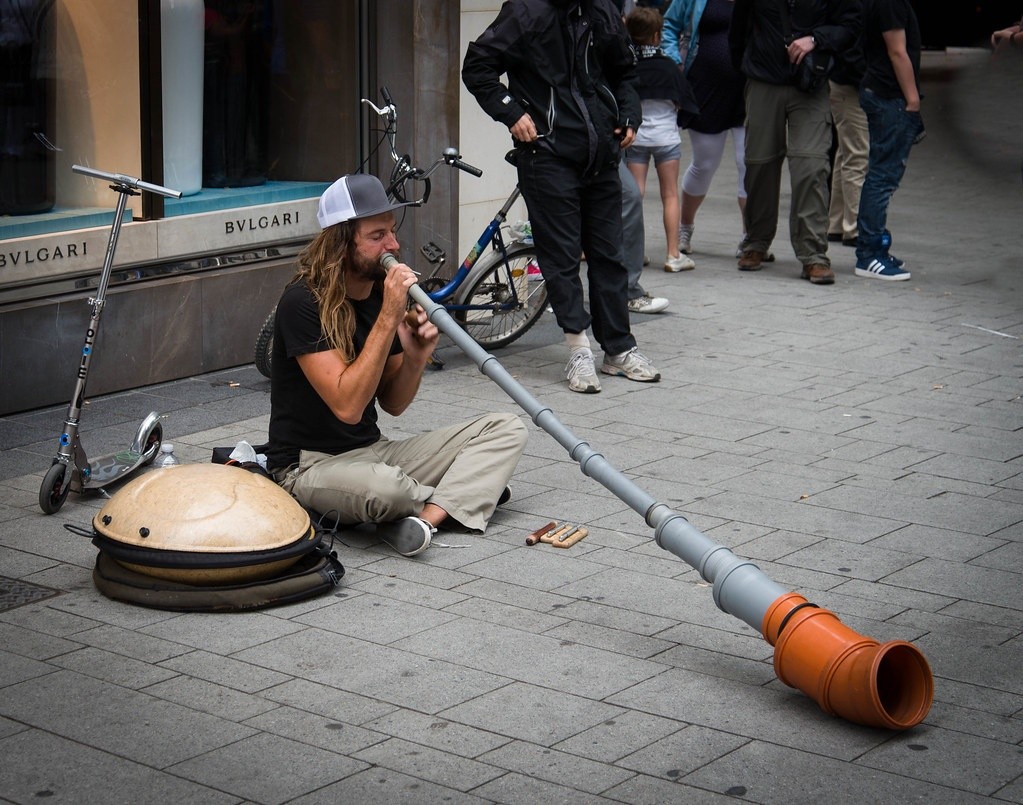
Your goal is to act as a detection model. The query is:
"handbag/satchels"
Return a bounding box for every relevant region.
[797,50,835,94]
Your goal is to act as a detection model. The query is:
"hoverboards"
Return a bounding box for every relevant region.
[37,164,184,515]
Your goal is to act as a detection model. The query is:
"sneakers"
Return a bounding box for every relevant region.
[738,249,763,268]
[666,252,696,271]
[739,239,775,262]
[800,262,835,283]
[601,346,660,382]
[564,351,603,392]
[677,222,695,251]
[853,254,910,281]
[626,294,668,312]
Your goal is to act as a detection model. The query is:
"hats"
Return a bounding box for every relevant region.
[317,173,421,230]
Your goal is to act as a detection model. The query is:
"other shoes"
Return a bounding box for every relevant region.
[828,231,843,242]
[497,486,511,505]
[841,235,860,245]
[375,516,437,555]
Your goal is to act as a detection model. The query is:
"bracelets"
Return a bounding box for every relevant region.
[1008,32,1018,48]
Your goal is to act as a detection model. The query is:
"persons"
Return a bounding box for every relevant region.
[825,0,927,283]
[661,0,760,254]
[729,0,855,285]
[621,6,698,274]
[265,173,529,557]
[462,0,661,394]
[991,20,1023,75]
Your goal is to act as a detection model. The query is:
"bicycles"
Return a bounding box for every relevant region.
[252,84,548,383]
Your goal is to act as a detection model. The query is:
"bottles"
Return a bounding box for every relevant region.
[153,442,182,472]
